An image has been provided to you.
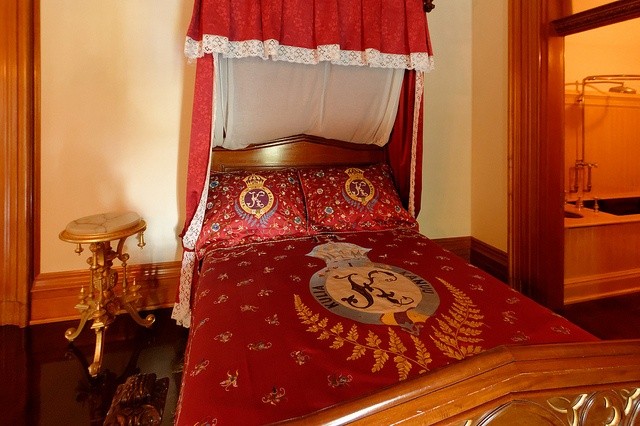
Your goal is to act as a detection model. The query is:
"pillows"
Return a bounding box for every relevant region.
[297,160,419,236]
[195,167,311,261]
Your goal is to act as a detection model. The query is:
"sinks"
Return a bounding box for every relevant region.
[564,210,584,219]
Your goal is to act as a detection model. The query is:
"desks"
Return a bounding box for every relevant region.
[58,211,156,382]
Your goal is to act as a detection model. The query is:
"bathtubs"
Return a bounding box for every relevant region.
[567,197,640,215]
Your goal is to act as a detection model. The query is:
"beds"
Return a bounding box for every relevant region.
[174,132,637,425]
